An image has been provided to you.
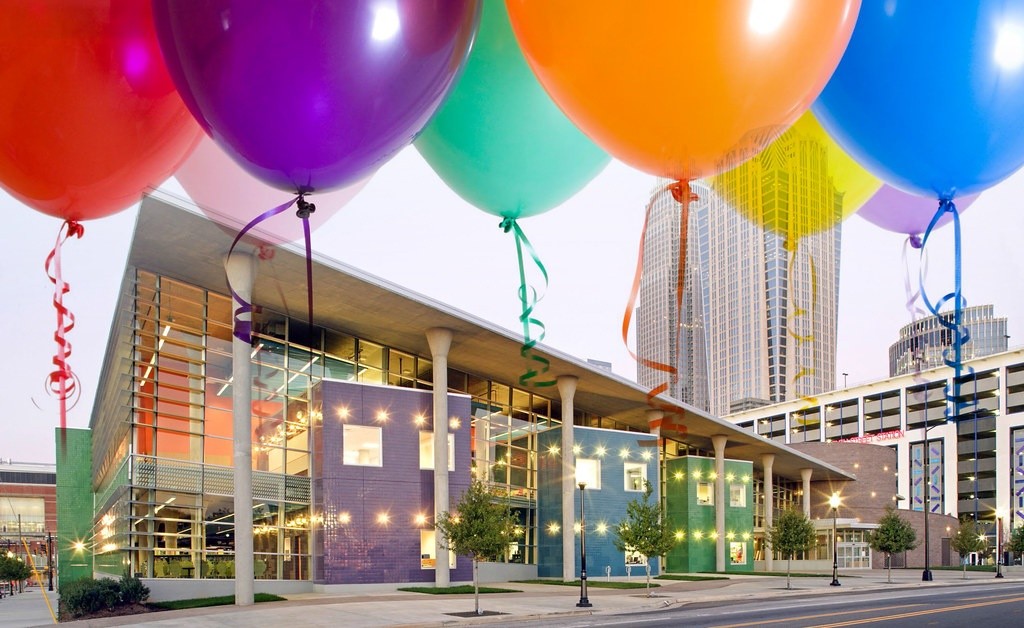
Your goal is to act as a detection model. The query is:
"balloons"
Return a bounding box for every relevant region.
[409,0,615,236]
[169,134,374,264]
[810,0,1024,214]
[503,0,864,206]
[0,0,207,236]
[856,186,982,247]
[697,115,883,258]
[149,0,484,221]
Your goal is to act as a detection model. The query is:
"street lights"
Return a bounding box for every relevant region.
[922,408,989,581]
